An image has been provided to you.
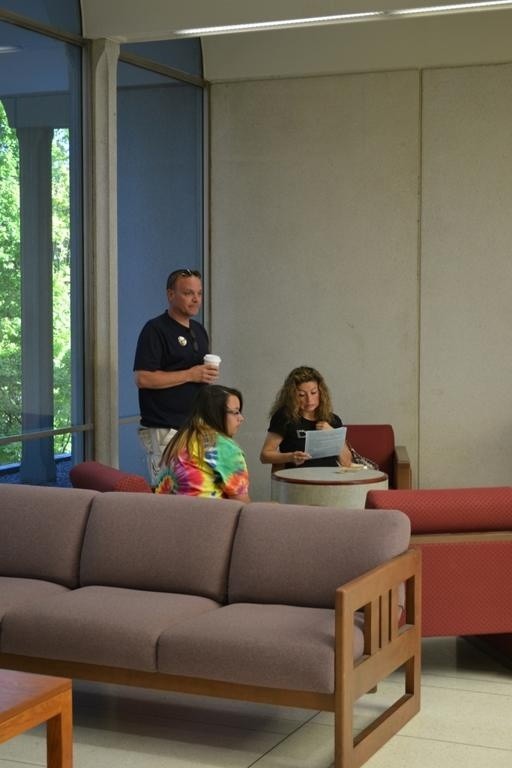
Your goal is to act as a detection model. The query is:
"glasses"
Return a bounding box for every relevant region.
[169,269,201,284]
[227,409,240,417]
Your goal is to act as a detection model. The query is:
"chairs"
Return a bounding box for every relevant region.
[69,461,152,492]
[364,485,512,672]
[337,422,411,490]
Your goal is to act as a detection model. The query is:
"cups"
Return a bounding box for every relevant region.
[203,354,222,366]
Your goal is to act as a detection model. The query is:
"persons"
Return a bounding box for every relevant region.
[149,382,255,498]
[132,263,225,499]
[256,361,361,467]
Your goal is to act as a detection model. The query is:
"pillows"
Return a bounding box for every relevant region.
[226,502,413,610]
[2,480,95,590]
[79,486,243,601]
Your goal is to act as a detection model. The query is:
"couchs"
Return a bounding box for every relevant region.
[1,548,420,767]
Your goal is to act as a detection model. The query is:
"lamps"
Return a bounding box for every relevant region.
[80,0,512,46]
